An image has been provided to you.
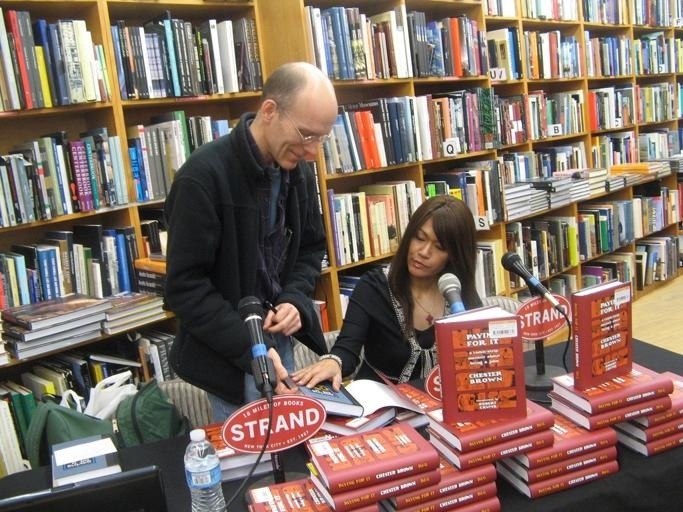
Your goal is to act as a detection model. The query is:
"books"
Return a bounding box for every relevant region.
[0,331,180,489]
[304,6,507,319]
[1,4,264,361]
[313,299,330,333]
[192,279,683,512]
[483,1,683,290]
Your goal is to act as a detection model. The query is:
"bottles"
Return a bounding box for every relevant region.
[182,428,230,512]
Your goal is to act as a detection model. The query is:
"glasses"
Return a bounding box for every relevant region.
[279,106,333,144]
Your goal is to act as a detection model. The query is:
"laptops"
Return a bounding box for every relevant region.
[0,464,168,512]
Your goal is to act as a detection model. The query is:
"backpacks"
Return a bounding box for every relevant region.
[24,378,191,470]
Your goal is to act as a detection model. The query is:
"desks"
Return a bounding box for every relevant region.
[0,336,683,510]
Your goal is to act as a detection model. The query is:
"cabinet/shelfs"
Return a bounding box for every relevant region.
[2,0,683,368]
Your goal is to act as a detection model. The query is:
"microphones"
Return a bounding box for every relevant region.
[500,251,565,315]
[437,271,466,315]
[237,296,277,403]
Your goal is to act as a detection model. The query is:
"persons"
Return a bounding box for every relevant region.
[288,194,482,391]
[164,61,338,423]
